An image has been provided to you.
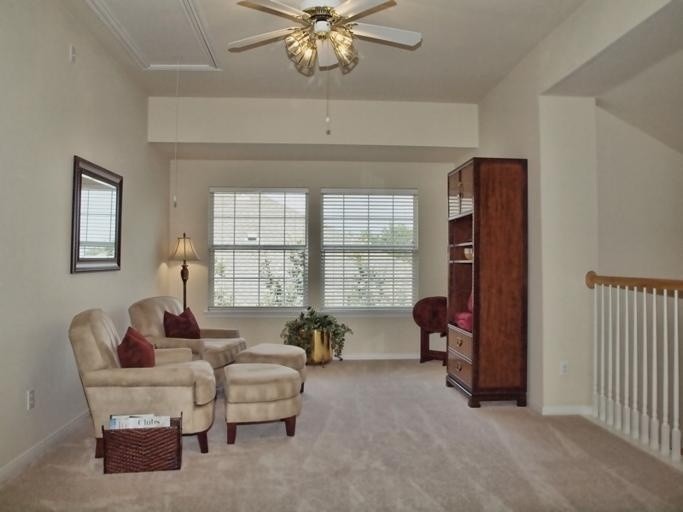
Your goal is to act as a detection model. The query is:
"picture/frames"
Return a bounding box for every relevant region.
[69,153,123,274]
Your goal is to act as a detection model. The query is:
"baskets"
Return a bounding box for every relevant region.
[101,411,183,474]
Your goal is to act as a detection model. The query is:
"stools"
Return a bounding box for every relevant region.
[220,360,303,446]
[243,341,308,396]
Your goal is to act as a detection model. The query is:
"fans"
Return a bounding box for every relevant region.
[225,1,426,52]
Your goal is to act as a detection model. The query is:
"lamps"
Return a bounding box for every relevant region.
[166,231,201,312]
[283,1,361,123]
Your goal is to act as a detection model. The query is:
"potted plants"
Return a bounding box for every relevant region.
[279,303,355,369]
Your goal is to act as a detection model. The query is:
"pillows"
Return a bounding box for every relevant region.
[116,325,156,369]
[162,303,201,339]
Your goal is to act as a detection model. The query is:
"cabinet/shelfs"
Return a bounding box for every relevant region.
[444,157,529,409]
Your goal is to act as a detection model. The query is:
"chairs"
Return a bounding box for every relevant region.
[127,295,249,403]
[65,306,218,459]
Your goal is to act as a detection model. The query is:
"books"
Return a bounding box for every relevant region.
[448,195,473,217]
[108,414,172,431]
